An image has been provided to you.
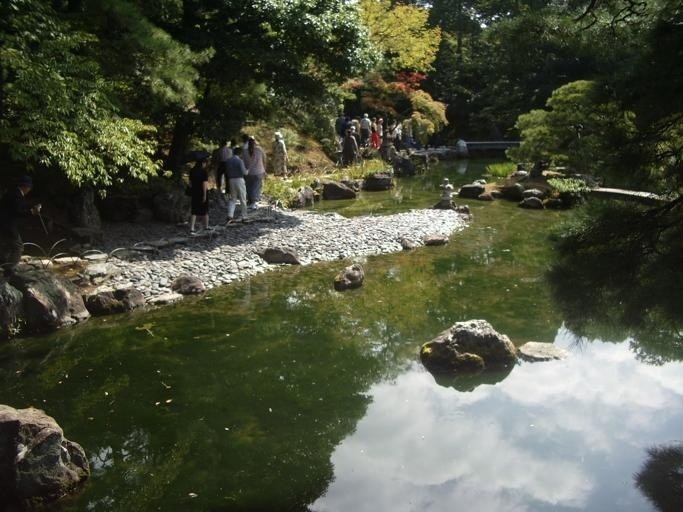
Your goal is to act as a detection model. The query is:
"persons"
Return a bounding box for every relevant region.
[224,147,249,223]
[239,133,257,148]
[0,174,45,277]
[270,130,290,180]
[242,136,266,209]
[335,110,412,167]
[213,138,232,201]
[185,152,212,236]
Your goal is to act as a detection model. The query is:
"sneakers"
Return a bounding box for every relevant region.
[188,230,197,235]
[202,227,215,233]
